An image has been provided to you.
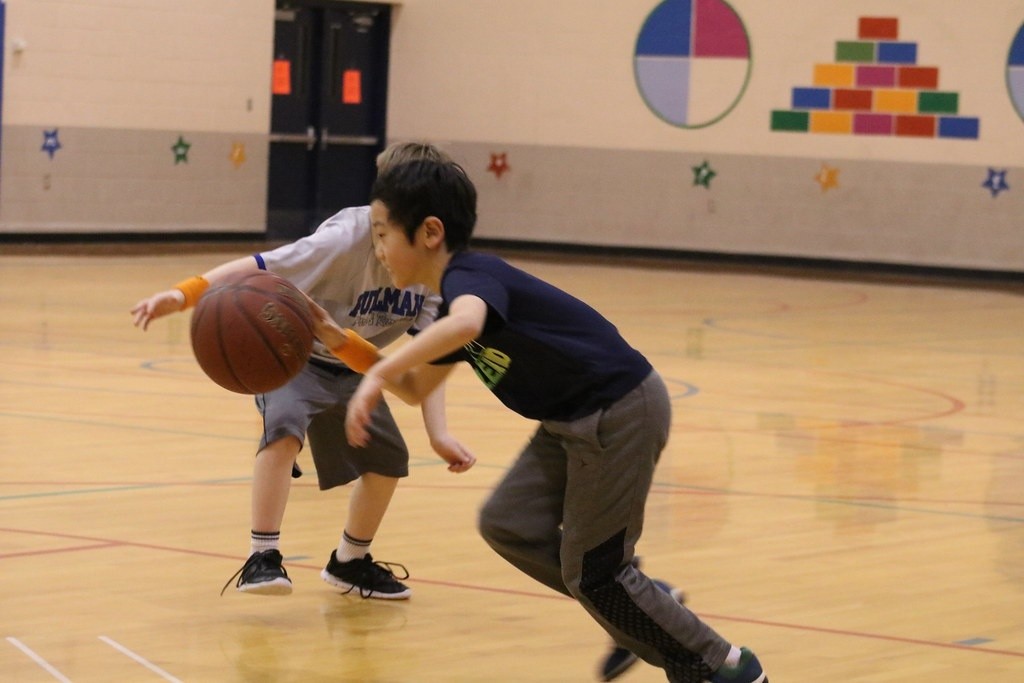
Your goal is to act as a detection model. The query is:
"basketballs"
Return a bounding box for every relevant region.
[189,267,316,395]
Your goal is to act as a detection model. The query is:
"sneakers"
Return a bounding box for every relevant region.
[236,551,293,597]
[704,648,768,683]
[321,550,412,601]
[604,579,682,680]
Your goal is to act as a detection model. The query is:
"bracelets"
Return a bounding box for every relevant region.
[328,328,380,374]
[174,275,211,312]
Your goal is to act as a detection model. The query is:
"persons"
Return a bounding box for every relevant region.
[132,141,477,603]
[299,157,768,683]
